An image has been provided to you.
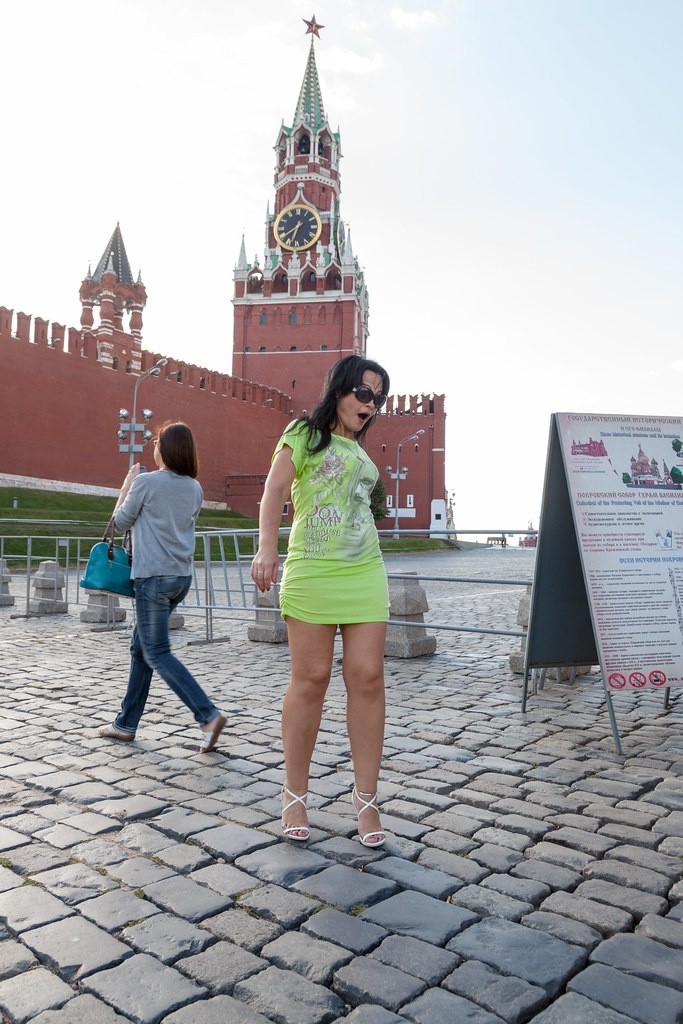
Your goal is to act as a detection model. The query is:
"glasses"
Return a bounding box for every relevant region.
[349,386,389,410]
[152,440,161,445]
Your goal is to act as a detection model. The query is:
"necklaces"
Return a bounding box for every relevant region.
[331,426,367,466]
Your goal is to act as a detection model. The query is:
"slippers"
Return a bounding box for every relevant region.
[96,722,134,740]
[198,716,227,753]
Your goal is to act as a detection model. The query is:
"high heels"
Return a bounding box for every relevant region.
[351,786,386,847]
[280,784,312,841]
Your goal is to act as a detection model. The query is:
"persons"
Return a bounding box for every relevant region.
[252,350,397,854]
[98,419,230,756]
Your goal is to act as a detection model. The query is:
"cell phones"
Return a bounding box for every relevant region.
[140,466,146,473]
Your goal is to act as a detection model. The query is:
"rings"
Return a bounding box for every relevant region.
[257,570,264,573]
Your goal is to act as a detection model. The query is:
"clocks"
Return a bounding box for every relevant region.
[273,203,323,252]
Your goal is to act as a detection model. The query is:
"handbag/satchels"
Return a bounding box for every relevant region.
[79,514,136,600]
